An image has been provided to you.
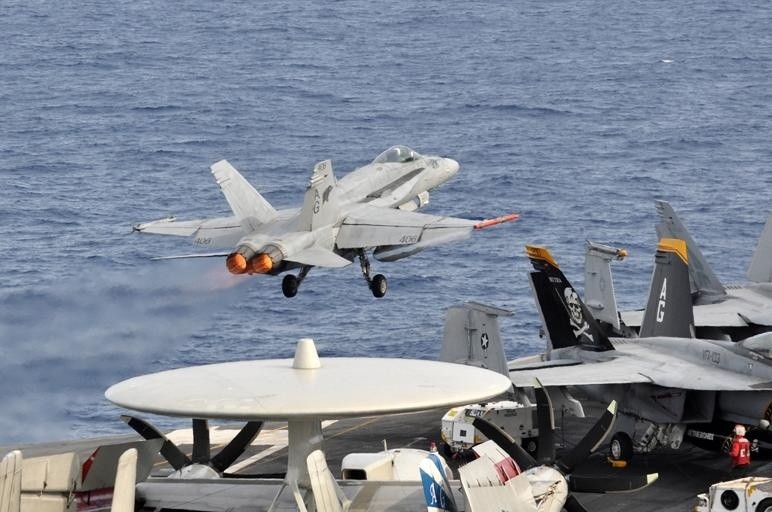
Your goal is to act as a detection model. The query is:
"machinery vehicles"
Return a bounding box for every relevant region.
[693,474,772,512]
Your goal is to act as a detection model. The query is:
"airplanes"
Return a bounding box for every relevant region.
[0,336,665,512]
[128,143,524,302]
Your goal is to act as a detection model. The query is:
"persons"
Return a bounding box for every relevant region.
[725,425,752,480]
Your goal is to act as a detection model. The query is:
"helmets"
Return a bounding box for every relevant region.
[733,424,747,436]
